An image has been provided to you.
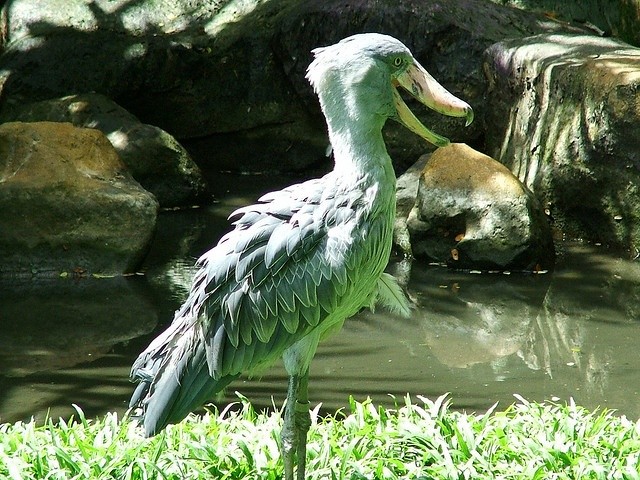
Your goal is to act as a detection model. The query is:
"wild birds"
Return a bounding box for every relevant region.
[127,29,477,480]
